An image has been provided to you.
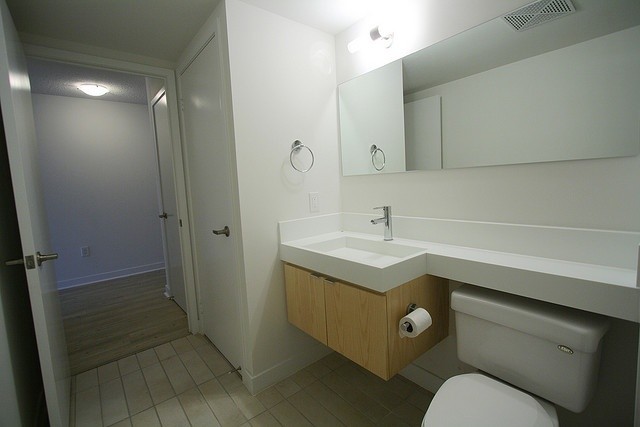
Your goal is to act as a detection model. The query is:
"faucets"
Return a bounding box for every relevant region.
[371,206,394,241]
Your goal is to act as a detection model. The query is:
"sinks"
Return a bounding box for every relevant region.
[298,234,431,270]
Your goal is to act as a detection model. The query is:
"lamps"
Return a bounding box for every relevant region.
[76,82,112,99]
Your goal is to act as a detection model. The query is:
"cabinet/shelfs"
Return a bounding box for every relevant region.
[282,262,449,381]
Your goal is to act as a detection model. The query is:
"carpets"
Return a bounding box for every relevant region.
[58,268,192,377]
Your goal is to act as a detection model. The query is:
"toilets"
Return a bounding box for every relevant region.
[419,287,611,427]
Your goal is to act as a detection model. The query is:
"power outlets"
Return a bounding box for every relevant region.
[309,192,320,212]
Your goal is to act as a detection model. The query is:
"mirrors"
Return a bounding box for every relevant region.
[337,57,405,174]
